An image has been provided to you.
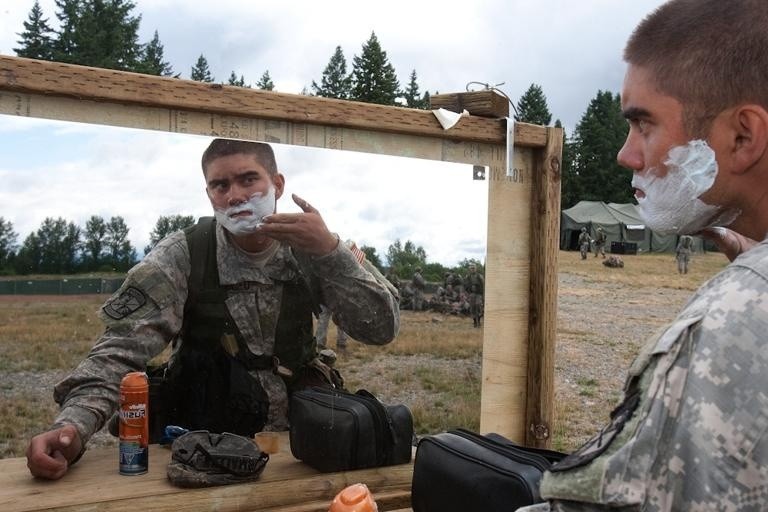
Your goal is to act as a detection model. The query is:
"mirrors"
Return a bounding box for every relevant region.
[2,111,490,512]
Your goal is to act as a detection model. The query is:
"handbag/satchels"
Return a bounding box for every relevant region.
[287,384,414,474]
[410,427,571,512]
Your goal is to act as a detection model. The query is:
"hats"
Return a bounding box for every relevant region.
[168,429,269,488]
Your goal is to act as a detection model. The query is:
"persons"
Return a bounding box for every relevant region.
[26,138,400,485]
[671,233,694,272]
[576,225,607,260]
[385,264,484,326]
[316,303,348,354]
[542,1,767,511]
